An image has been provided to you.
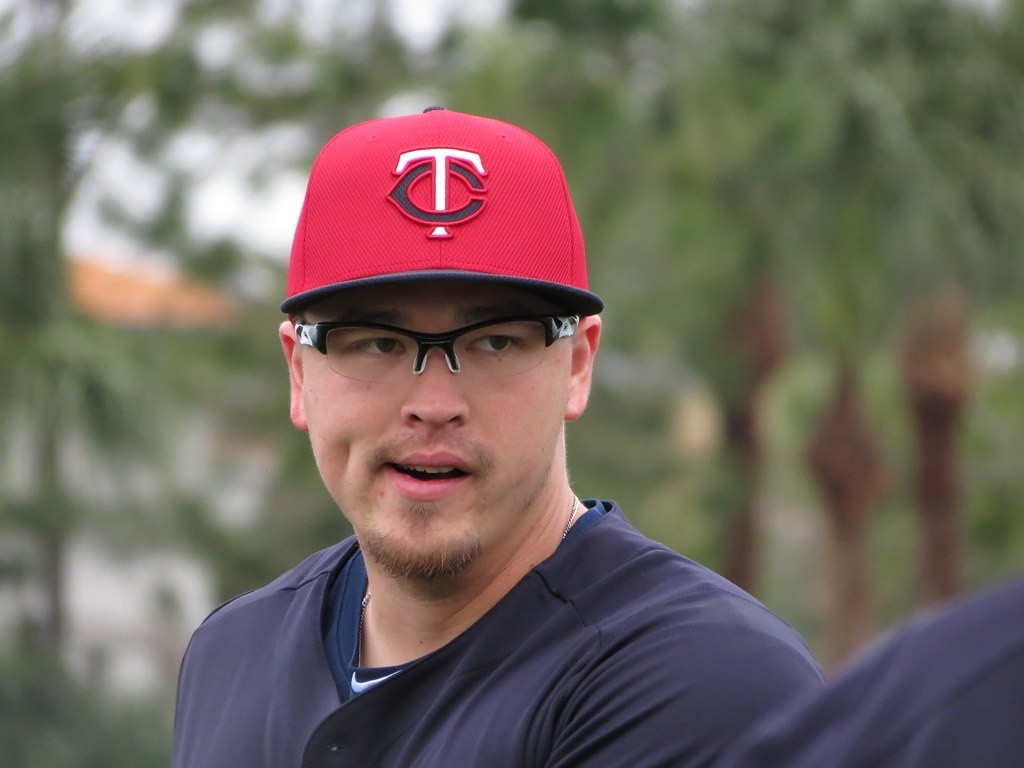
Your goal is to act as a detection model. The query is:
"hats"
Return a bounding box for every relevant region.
[281,106,604,317]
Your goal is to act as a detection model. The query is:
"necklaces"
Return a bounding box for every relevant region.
[356,495,583,659]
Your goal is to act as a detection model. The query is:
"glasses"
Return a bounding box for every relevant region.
[295,313,579,384]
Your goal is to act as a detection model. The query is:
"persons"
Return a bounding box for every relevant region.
[711,570,1024,768]
[174,106,827,768]
